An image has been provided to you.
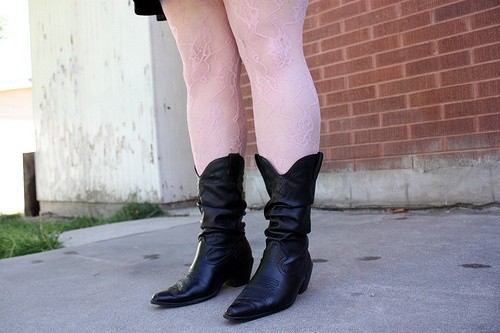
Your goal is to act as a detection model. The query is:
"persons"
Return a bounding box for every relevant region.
[134,1,323,320]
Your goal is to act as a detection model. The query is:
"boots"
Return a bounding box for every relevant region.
[150,153,255,307]
[223,152,325,321]
